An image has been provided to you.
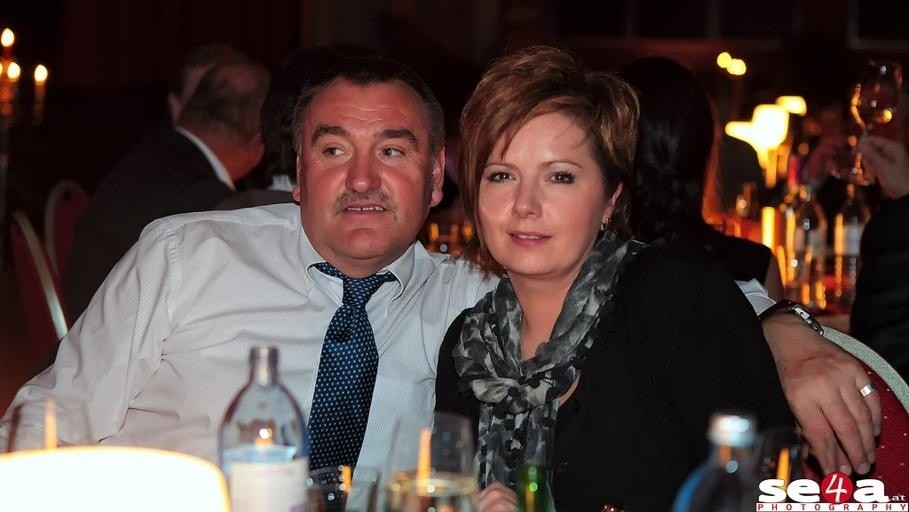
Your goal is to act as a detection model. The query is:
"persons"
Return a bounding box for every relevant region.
[66,4,908,377]
[429,39,808,512]
[2,52,885,512]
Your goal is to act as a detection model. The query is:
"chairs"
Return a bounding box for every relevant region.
[815,325,909,512]
[4,178,98,369]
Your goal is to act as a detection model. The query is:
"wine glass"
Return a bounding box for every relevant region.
[827,58,901,184]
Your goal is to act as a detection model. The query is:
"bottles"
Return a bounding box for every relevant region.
[676,412,763,512]
[516,461,551,510]
[220,346,311,511]
[787,173,863,308]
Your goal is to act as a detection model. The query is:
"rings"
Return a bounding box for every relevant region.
[860,378,876,403]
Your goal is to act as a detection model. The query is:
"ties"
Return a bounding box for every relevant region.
[301,259,400,511]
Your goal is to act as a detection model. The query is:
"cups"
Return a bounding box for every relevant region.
[312,469,380,512]
[372,412,481,510]
